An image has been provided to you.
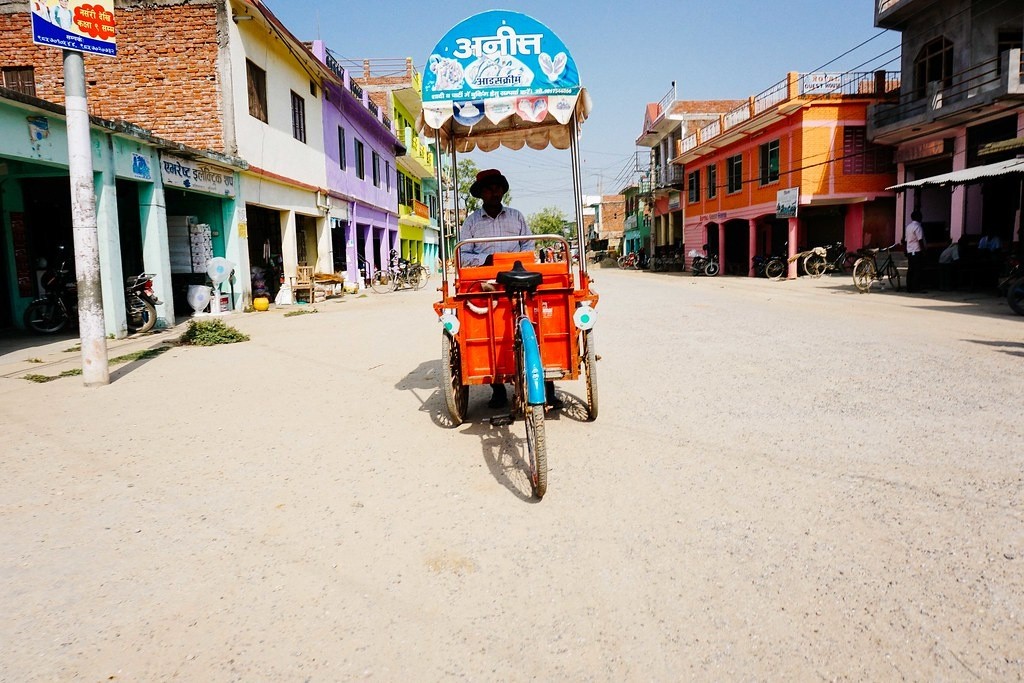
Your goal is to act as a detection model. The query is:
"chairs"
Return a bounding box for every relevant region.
[289,266,314,304]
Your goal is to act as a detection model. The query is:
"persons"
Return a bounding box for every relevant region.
[905,211,927,293]
[938,230,1003,292]
[546,242,565,263]
[461,169,538,266]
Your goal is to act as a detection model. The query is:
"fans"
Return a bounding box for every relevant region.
[188,285,211,316]
[206,256,233,316]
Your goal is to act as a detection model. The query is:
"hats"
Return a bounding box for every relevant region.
[470,169,509,198]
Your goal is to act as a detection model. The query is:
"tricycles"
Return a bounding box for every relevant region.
[417,10,603,500]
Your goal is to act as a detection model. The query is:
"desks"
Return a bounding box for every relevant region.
[317,278,346,296]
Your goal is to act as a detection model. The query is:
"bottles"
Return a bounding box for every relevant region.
[280,273,284,283]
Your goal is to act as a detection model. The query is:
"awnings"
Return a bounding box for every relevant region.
[885,158,1024,193]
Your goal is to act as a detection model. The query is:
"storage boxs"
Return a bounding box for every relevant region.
[209,293,231,312]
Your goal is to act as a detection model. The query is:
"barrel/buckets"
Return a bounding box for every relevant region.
[380,276,389,285]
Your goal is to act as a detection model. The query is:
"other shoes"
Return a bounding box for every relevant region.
[547,396,562,409]
[488,392,507,408]
[921,289,928,293]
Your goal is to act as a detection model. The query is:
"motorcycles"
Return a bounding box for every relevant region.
[996,258,1024,317]
[689,244,719,277]
[23,240,163,335]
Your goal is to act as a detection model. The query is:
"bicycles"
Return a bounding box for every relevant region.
[616,249,684,272]
[851,243,902,294]
[759,241,862,281]
[370,258,428,294]
